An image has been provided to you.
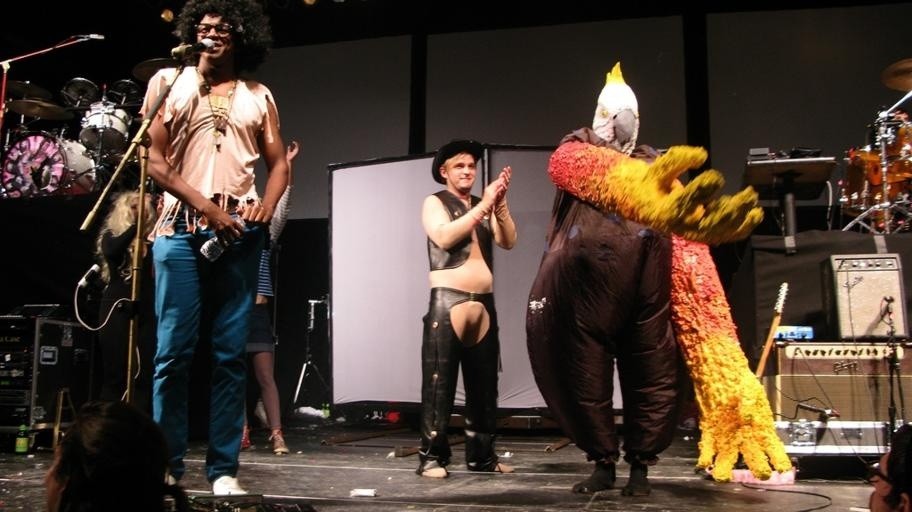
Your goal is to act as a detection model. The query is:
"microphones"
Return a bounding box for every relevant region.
[185,37,214,58]
[89,32,106,42]
[797,402,841,418]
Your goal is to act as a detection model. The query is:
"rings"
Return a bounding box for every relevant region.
[246,198,254,206]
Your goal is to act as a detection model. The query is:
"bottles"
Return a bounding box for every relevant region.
[319,402,331,418]
[14,425,29,455]
[197,217,247,262]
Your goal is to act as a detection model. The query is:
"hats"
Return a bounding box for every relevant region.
[429,137,485,185]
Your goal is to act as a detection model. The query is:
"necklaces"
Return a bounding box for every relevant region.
[205,77,237,146]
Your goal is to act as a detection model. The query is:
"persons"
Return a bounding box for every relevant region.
[240,140,299,453]
[136,1,290,496]
[869,423,911,511]
[46,399,191,512]
[416,138,518,478]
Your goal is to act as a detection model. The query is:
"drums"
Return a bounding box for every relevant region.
[0,132,96,197]
[79,100,133,152]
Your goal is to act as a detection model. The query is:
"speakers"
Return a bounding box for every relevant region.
[819,252,911,341]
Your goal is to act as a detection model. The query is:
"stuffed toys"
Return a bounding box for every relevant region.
[525,59,793,498]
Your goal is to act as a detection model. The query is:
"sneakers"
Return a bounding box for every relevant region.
[419,464,449,478]
[467,460,516,473]
[240,428,253,449]
[164,473,179,497]
[268,429,290,454]
[210,475,252,497]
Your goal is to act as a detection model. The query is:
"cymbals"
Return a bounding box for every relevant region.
[6,79,53,101]
[134,58,179,80]
[882,57,911,92]
[5,95,77,121]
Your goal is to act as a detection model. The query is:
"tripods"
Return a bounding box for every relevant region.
[840,147,912,235]
[278,335,346,427]
[52,141,123,193]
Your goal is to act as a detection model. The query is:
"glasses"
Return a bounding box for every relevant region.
[866,458,895,486]
[193,22,242,33]
